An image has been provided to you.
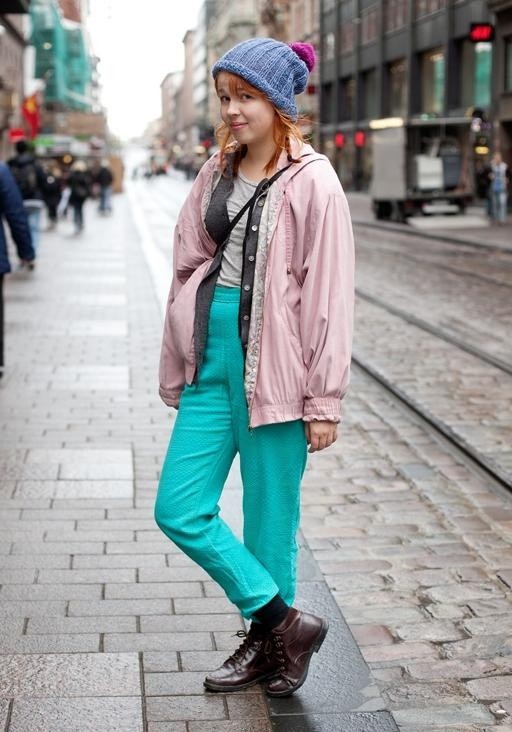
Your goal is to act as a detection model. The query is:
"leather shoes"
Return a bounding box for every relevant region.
[269,608,328,696]
[204,631,278,691]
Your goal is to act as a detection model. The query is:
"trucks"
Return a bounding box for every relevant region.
[370,118,475,223]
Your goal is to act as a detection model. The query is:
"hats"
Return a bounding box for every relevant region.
[211,38,316,125]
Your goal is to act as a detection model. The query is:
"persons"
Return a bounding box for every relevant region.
[37,164,62,217]
[92,158,115,216]
[0,159,38,378]
[481,151,510,226]
[63,160,92,230]
[8,159,35,200]
[15,140,40,194]
[152,33,356,698]
